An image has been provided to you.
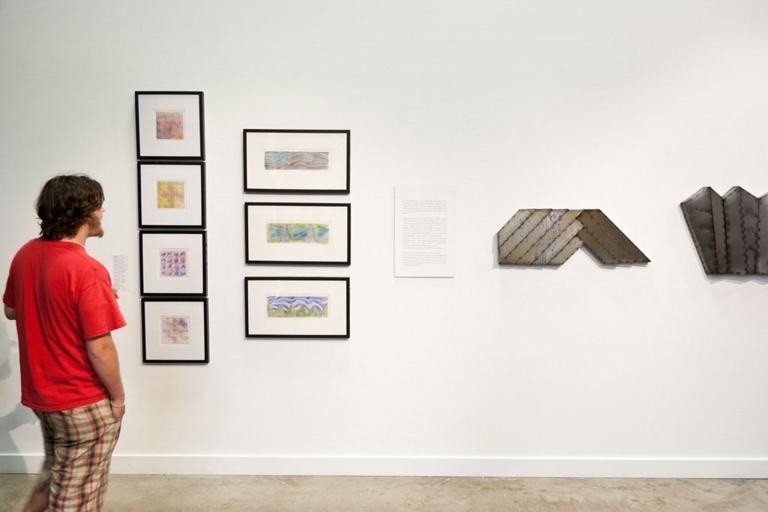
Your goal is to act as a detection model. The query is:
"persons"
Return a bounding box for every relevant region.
[2,170,129,511]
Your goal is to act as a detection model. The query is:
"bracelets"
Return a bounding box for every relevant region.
[109,399,128,410]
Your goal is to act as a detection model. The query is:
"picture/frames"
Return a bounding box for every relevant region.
[245,202,350,265]
[136,91,208,363]
[244,129,350,193]
[245,277,350,339]
